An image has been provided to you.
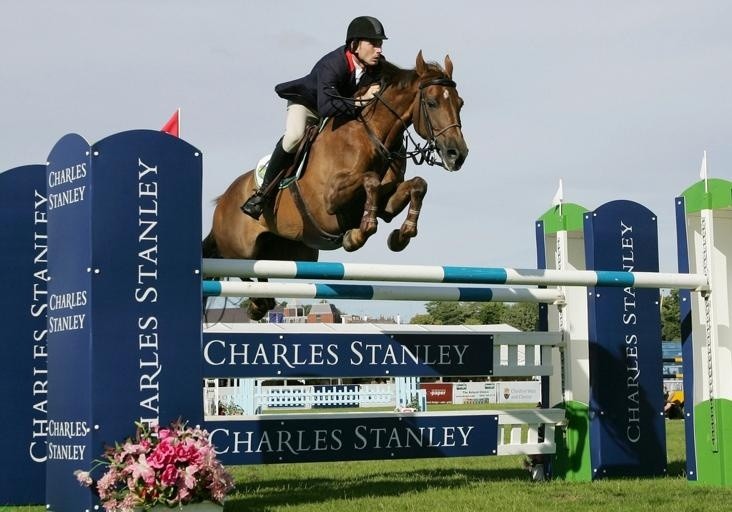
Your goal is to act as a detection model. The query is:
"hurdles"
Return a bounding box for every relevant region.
[0,129,732,512]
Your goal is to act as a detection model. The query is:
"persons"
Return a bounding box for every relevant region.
[242,16,392,221]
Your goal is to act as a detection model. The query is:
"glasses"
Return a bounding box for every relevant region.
[357,38,382,47]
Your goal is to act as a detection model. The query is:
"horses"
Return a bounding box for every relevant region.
[201,47,470,330]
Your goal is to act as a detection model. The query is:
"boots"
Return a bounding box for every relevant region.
[240,135,297,221]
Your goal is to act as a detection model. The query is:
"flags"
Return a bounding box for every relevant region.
[160,110,180,140]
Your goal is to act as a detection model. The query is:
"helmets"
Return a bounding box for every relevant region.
[346,16,388,43]
